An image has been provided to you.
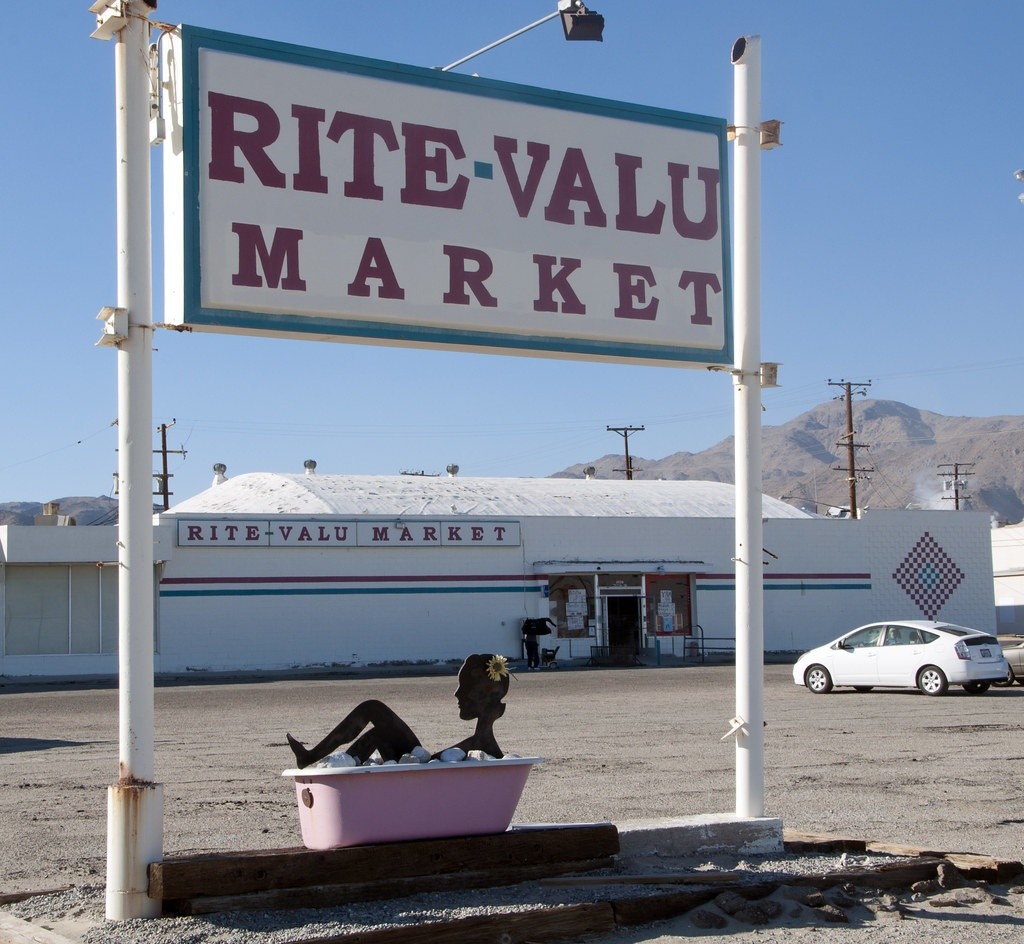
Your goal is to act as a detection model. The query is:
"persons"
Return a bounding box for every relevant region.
[285,654,510,770]
[523,617,542,671]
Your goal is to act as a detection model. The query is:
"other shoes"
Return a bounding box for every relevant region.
[534,667,540,670]
[528,667,532,671]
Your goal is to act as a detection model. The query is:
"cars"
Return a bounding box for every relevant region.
[990,639,1024,687]
[793,620,1007,696]
